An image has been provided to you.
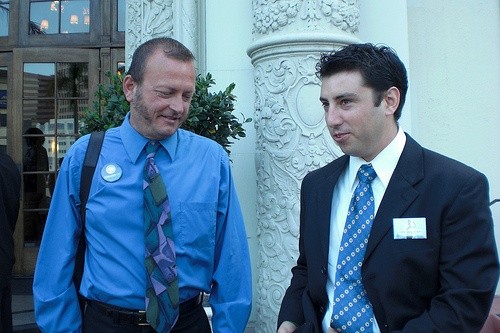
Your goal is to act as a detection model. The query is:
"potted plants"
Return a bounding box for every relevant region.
[78,68,252,333]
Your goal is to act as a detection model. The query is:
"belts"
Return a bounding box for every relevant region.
[88,293,202,327]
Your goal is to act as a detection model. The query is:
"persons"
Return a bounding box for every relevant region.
[31,37,253,333]
[274,44,500,333]
[0,146,20,333]
[23,127,49,243]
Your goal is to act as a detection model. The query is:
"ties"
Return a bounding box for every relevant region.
[143,141,179,332]
[330,164,377,333]
